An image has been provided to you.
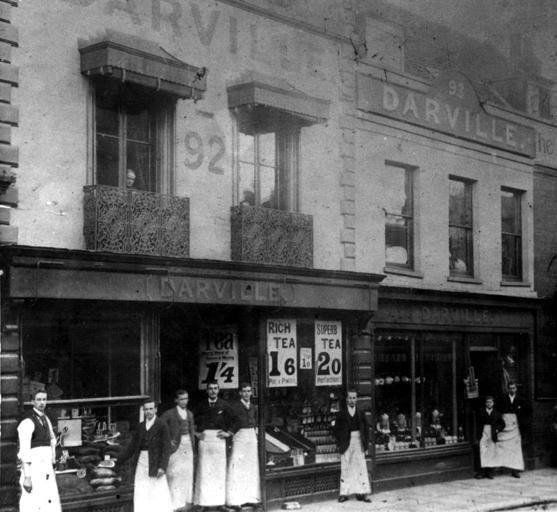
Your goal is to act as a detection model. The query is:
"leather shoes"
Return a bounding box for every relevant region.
[471,468,520,480]
[336,495,373,503]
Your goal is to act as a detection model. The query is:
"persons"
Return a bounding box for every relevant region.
[15,389,62,511]
[333,387,372,502]
[126,168,136,190]
[494,379,524,478]
[473,394,506,479]
[159,389,194,512]
[190,378,235,511]
[225,383,263,512]
[114,398,172,512]
